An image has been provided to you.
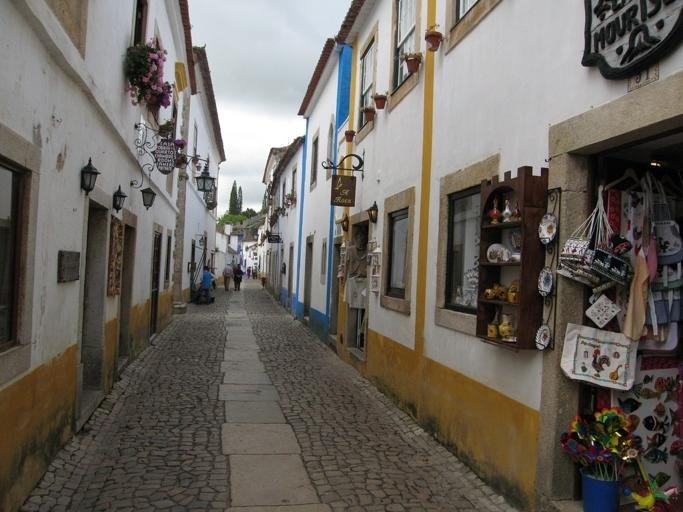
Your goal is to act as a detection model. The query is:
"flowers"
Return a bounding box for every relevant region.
[126,38,175,110]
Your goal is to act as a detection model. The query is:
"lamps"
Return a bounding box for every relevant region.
[366,200,378,223]
[131,163,156,211]
[81,156,102,196]
[113,185,128,213]
[176,145,216,192]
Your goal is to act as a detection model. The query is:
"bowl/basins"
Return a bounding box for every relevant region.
[511,253,521,264]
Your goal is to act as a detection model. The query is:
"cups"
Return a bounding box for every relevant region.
[485,289,497,299]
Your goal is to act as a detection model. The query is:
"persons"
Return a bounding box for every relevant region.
[246,265,257,279]
[223,263,243,292]
[194,266,216,305]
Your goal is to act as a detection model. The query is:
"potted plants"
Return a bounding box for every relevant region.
[358,93,388,121]
[404,23,446,73]
[345,129,357,142]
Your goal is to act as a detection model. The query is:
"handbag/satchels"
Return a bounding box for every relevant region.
[650,265,683,292]
[585,294,620,329]
[637,320,680,356]
[654,219,683,266]
[644,287,683,325]
[559,322,640,392]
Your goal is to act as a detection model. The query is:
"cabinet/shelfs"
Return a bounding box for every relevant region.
[475,164,549,351]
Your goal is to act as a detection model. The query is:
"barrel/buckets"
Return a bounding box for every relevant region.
[579,466,626,512]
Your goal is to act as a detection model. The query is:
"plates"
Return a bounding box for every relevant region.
[487,243,512,264]
[538,214,557,245]
[537,267,555,296]
[535,325,552,349]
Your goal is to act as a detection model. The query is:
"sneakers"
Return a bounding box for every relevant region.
[557,233,634,293]
